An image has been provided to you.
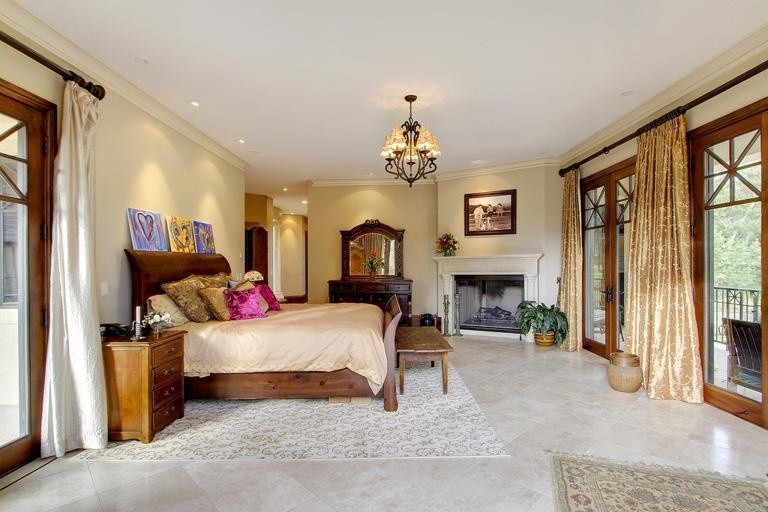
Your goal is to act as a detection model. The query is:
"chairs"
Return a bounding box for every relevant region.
[720,316,762,393]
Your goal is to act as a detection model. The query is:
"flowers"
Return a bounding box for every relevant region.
[433,232,460,258]
[141,310,172,329]
[362,249,386,272]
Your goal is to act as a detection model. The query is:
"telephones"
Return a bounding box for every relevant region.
[103,324,127,336]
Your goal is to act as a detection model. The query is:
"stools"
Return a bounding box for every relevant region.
[592,304,624,343]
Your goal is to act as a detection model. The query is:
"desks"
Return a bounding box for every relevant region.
[395,325,455,395]
[326,279,415,327]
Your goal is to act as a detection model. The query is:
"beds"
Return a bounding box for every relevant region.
[121,247,405,413]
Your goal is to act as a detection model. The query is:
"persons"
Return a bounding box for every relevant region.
[474,203,504,231]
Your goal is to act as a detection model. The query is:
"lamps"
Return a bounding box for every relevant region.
[243,269,264,283]
[379,94,442,191]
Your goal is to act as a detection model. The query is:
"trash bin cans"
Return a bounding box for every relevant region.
[421,314,434,326]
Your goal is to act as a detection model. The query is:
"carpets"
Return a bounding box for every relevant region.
[542,447,768,511]
[71,358,513,467]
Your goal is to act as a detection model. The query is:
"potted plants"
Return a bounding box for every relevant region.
[512,298,569,348]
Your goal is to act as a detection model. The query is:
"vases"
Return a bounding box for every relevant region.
[368,271,377,281]
[606,351,645,394]
[443,250,449,257]
[153,322,160,339]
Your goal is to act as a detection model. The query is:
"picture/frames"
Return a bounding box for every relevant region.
[462,187,518,238]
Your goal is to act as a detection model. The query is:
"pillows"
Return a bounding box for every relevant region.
[143,271,282,329]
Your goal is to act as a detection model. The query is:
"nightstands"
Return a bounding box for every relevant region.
[99,329,189,446]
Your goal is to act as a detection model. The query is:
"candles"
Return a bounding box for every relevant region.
[134,305,142,323]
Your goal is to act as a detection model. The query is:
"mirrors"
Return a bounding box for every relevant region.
[338,218,406,279]
